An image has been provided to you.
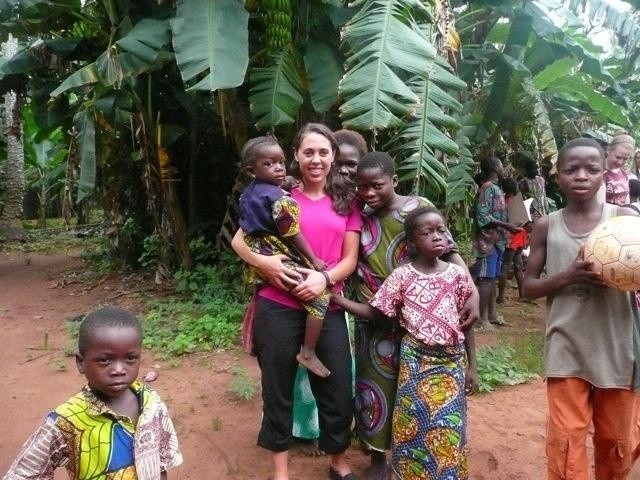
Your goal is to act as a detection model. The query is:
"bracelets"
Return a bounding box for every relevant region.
[321,270,331,286]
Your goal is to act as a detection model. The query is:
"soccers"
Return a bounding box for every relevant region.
[583,216,640,291]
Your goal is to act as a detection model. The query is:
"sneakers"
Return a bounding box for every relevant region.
[329,465,358,480]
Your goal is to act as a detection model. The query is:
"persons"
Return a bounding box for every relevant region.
[473,154,551,333]
[629,179,640,210]
[230,121,363,480]
[627,149,640,181]
[333,129,368,176]
[1,306,184,480]
[350,150,481,480]
[237,134,331,380]
[597,134,631,205]
[329,205,480,480]
[518,136,640,479]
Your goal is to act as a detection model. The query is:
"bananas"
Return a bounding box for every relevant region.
[262,0,293,58]
